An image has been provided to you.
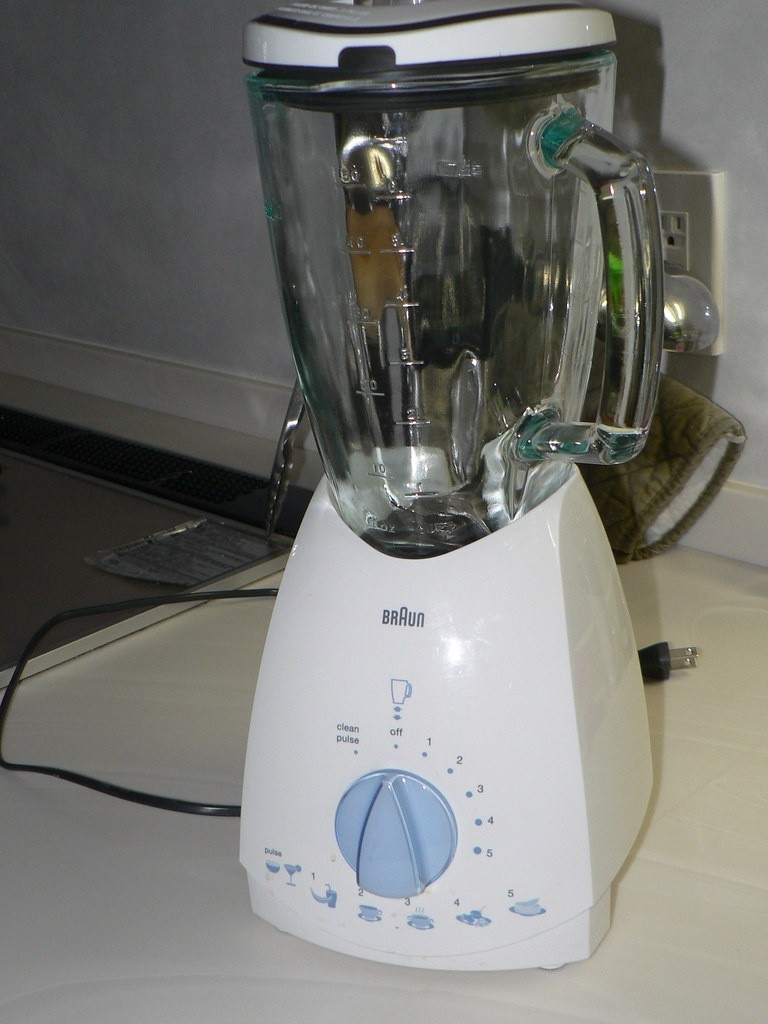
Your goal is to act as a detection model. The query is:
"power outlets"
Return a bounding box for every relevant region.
[650,168,731,356]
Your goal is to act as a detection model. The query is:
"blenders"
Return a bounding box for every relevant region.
[235,0,670,970]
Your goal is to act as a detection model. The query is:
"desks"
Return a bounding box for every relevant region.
[0,541,767,1024]
